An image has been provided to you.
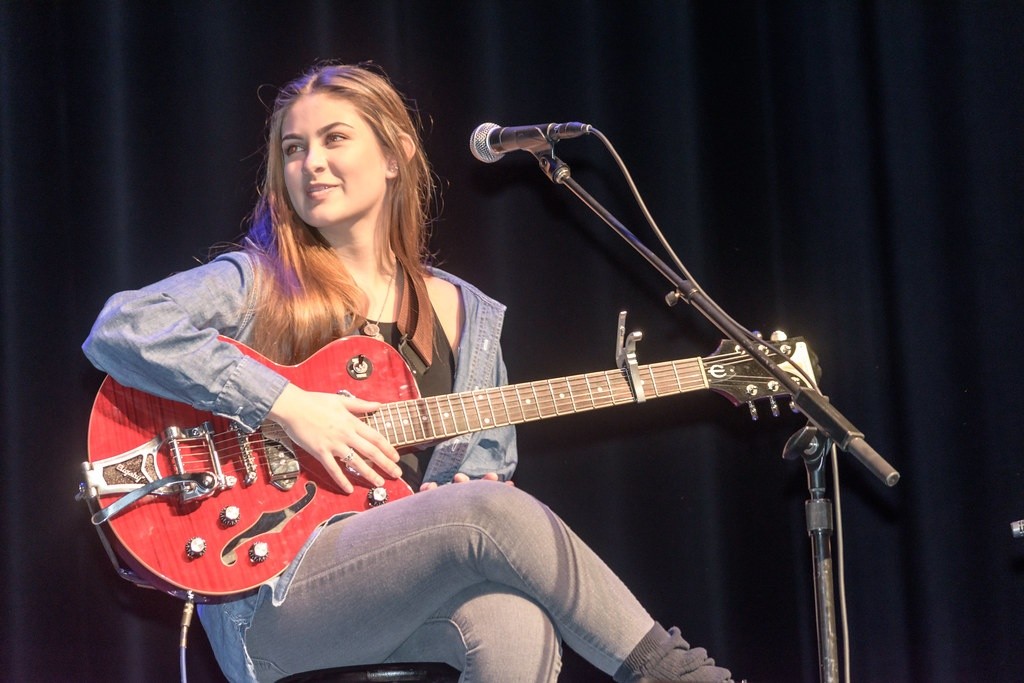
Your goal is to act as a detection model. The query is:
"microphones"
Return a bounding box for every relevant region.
[469,122,593,164]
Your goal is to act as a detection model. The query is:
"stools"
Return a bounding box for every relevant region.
[276,662,459,683]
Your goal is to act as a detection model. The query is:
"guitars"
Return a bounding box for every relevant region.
[82,323,826,606]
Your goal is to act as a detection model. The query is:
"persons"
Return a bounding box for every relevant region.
[79,59,741,682]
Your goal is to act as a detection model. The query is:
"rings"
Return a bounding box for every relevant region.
[340,448,355,463]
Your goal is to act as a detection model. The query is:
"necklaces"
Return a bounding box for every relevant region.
[360,259,398,336]
